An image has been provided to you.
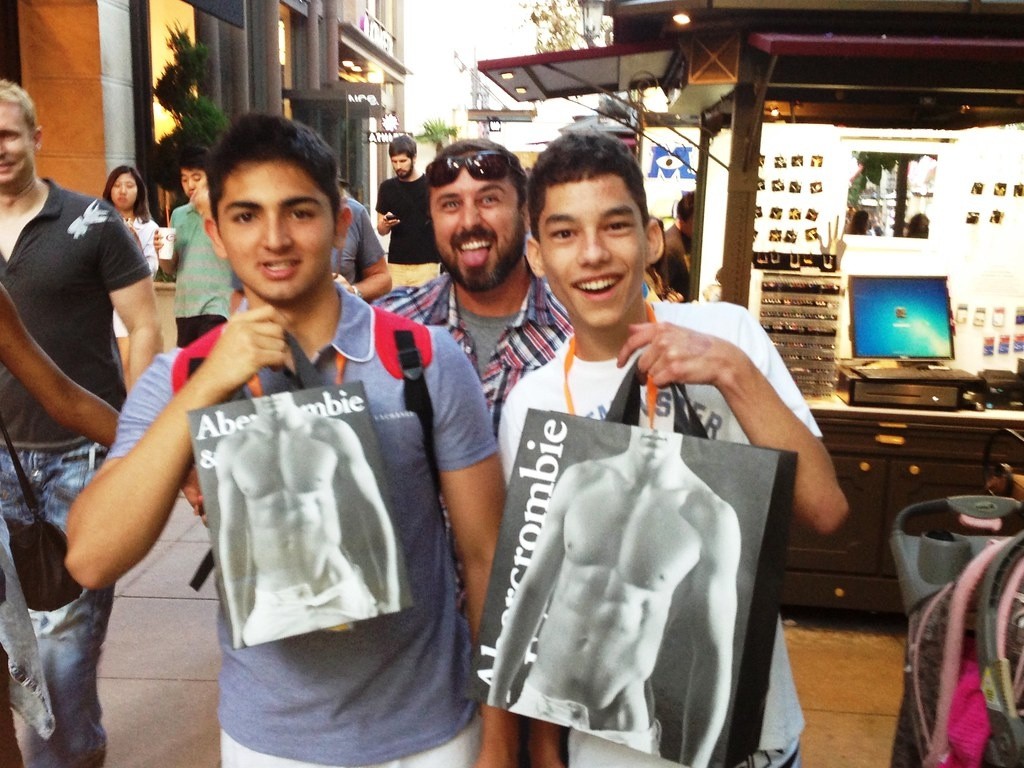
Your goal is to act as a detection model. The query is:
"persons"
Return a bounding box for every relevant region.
[153,148,233,349]
[0,283,208,768]
[0,79,167,768]
[375,135,443,288]
[644,191,696,301]
[216,393,399,648]
[103,166,158,390]
[65,117,520,767]
[230,170,392,316]
[499,137,848,768]
[485,426,741,768]
[844,205,929,239]
[373,139,574,427]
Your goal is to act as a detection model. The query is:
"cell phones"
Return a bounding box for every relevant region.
[386,215,398,220]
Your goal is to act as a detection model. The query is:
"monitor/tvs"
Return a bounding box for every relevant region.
[848,275,954,368]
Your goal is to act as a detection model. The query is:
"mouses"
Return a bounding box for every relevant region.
[960,401,984,412]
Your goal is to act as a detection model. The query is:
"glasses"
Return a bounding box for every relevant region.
[425,150,525,188]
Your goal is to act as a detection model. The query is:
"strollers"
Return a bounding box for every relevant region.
[889,497,1024,768]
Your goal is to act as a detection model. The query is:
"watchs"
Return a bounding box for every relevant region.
[352,285,363,298]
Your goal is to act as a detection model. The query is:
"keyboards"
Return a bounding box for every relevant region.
[850,367,985,384]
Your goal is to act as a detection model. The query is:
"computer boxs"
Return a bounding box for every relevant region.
[834,366,959,410]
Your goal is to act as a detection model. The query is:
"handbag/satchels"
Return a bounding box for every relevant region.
[2,515,85,614]
[185,327,419,646]
[457,352,800,768]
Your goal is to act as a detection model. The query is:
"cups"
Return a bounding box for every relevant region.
[157,227,176,260]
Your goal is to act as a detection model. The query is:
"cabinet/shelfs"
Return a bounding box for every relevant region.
[777,394,1023,615]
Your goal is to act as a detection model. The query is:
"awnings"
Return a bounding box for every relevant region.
[743,30,1024,169]
[477,40,729,172]
